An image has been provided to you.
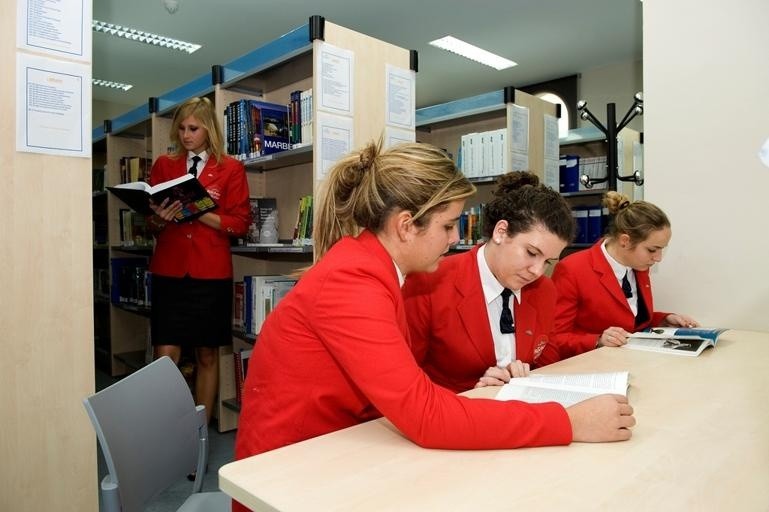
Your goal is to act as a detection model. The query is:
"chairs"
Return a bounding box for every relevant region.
[80,355,232,511]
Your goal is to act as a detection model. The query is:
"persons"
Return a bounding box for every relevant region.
[551,190,698,360]
[145,96,252,481]
[230,133,634,512]
[401,168,577,394]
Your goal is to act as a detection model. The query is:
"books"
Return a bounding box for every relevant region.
[456,203,486,245]
[119,208,153,247]
[105,174,219,224]
[120,156,152,184]
[237,194,313,246]
[494,370,631,409]
[462,127,510,177]
[559,154,609,190]
[224,88,313,158]
[118,262,153,307]
[572,205,611,243]
[233,274,297,336]
[621,325,731,358]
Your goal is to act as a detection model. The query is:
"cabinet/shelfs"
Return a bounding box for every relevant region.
[92,13,418,434]
[558,123,643,263]
[414,85,561,254]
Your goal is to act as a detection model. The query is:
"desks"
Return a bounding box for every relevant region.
[217,325,768,512]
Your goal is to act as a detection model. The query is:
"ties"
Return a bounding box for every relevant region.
[188,156,201,179]
[620,272,635,299]
[499,288,516,333]
